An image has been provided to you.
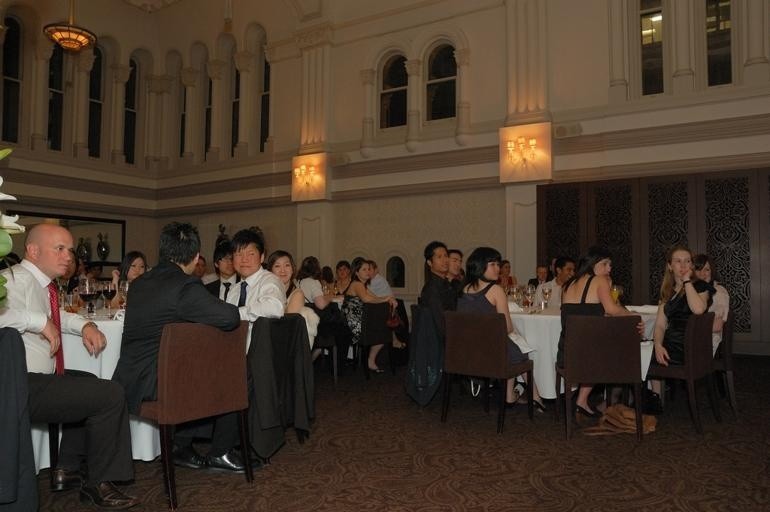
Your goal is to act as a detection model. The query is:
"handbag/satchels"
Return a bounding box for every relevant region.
[631,389,663,415]
[602,404,656,435]
[387,316,400,328]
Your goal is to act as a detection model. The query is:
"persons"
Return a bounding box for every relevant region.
[528,243,729,424]
[422,242,546,410]
[2,223,138,511]
[194,230,399,371]
[112,223,262,474]
[59,249,146,294]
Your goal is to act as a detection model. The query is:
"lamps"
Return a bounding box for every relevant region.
[294,165,316,184]
[505,135,537,165]
[43,0,99,56]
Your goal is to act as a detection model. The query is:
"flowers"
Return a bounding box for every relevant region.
[0,148,26,314]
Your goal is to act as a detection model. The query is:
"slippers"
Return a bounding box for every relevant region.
[504,396,527,409]
[533,397,547,413]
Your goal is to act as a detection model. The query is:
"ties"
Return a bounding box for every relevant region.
[48,283,64,376]
[238,282,248,307]
[223,283,231,303]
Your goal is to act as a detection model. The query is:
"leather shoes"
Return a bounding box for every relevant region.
[80,482,141,510]
[370,368,385,374]
[576,404,602,418]
[51,465,81,491]
[171,443,205,469]
[206,452,247,474]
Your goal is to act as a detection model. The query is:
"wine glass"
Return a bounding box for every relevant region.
[503,283,558,310]
[56,277,135,320]
[611,284,624,307]
[321,281,342,299]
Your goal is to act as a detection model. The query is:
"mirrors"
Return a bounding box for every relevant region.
[5,210,126,282]
